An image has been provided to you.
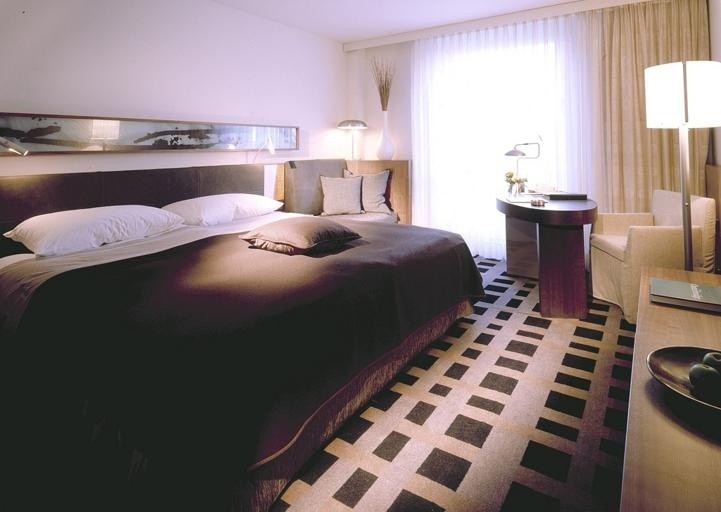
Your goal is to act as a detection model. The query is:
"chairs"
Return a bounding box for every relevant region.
[284,159,398,224]
[589,189,717,325]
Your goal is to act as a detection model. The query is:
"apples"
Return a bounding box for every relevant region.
[703,352,721,369]
[689,363,719,387]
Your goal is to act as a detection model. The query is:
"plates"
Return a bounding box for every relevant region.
[646,346,721,414]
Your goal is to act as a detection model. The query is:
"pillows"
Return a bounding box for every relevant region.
[320,168,392,215]
[238,217,362,255]
[3,193,285,259]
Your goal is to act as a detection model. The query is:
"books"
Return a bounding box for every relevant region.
[544,193,587,201]
[650,277,721,314]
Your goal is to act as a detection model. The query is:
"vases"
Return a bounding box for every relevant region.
[377,110,395,161]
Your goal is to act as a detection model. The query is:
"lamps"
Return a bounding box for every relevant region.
[0,137,29,157]
[252,134,276,164]
[505,143,540,195]
[338,120,369,159]
[644,60,721,271]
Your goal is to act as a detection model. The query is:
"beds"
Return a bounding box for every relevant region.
[0,165,484,512]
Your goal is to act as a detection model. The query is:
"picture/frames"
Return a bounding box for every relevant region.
[0,112,300,157]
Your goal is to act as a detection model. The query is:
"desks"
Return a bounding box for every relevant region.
[497,197,598,319]
[619,266,721,512]
